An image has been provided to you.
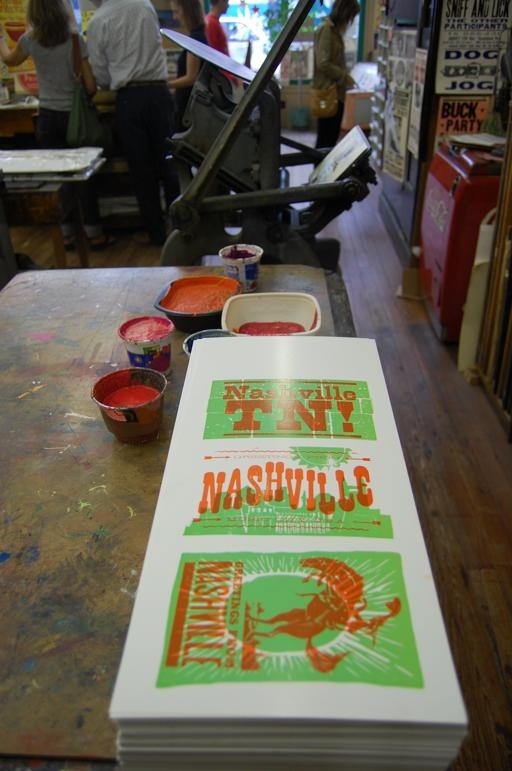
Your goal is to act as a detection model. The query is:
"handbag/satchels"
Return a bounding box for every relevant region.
[66,33,112,146]
[308,81,339,117]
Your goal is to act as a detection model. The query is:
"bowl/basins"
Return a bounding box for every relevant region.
[153,275,321,357]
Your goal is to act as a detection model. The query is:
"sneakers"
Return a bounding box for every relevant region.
[63,234,118,251]
[133,232,150,245]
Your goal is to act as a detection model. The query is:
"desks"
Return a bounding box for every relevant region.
[1,263,358,761]
[1,144,109,267]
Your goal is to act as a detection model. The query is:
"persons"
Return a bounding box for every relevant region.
[86,0,180,249]
[313,1,360,173]
[165,0,209,182]
[1,1,119,251]
[204,1,242,110]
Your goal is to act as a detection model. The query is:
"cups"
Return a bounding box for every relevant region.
[89,315,176,445]
[218,243,264,293]
